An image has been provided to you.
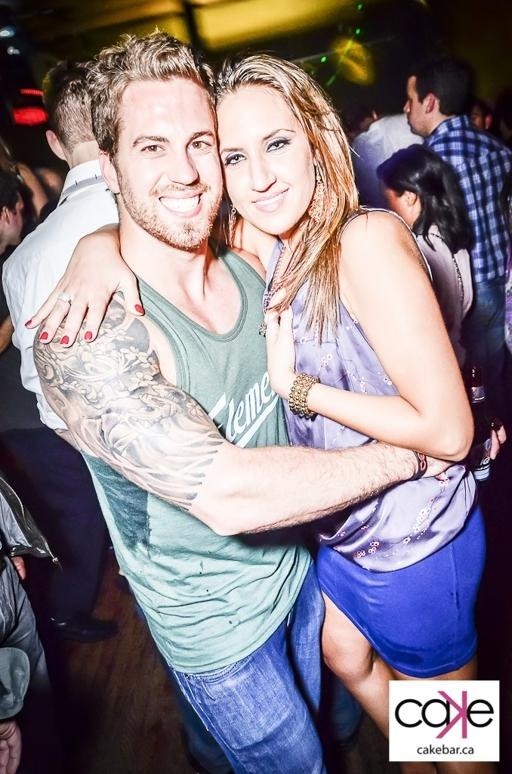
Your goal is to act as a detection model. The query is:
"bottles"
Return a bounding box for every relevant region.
[464,362,492,466]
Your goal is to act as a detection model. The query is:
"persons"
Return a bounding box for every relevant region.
[0,30,512,774]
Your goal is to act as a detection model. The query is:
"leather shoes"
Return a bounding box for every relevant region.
[39,610,119,650]
